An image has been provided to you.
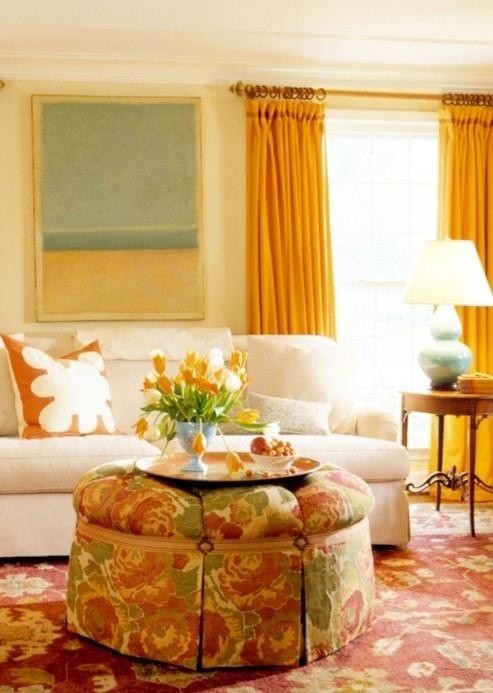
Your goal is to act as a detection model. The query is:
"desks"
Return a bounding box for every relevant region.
[399,383,493,542]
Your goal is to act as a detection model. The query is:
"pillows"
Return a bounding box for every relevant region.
[0,336,130,439]
[235,334,358,438]
[73,327,235,362]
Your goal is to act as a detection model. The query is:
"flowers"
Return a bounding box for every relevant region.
[127,341,272,454]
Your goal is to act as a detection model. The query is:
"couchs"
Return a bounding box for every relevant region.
[0,334,416,564]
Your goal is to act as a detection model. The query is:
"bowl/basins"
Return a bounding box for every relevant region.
[249,448,297,472]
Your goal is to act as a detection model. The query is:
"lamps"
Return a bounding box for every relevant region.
[403,239,493,392]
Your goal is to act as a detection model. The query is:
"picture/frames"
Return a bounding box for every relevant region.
[29,89,209,324]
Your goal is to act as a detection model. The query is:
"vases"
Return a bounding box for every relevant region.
[175,418,218,473]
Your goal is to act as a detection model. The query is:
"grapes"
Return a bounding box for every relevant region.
[262,439,294,456]
[263,471,271,478]
[289,467,296,474]
[246,470,253,477]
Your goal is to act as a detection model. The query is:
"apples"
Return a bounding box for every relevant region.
[251,437,266,455]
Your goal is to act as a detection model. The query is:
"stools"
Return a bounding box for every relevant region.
[63,454,380,674]
